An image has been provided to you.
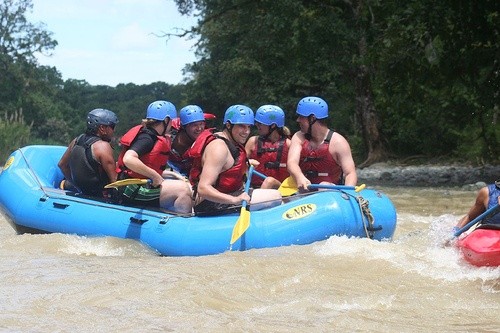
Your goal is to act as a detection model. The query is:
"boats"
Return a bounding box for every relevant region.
[0,144,398,258]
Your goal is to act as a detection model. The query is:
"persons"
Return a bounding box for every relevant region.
[57,108,120,196]
[445,181,500,247]
[116,96,358,217]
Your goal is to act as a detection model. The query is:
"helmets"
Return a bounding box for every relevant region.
[255,104,287,128]
[146,100,176,123]
[86,108,122,128]
[222,104,256,128]
[179,104,206,125]
[296,95,330,120]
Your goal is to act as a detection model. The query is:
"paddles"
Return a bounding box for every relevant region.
[103,177,174,189]
[277,174,367,197]
[253,169,267,179]
[452,203,499,237]
[229,163,255,245]
